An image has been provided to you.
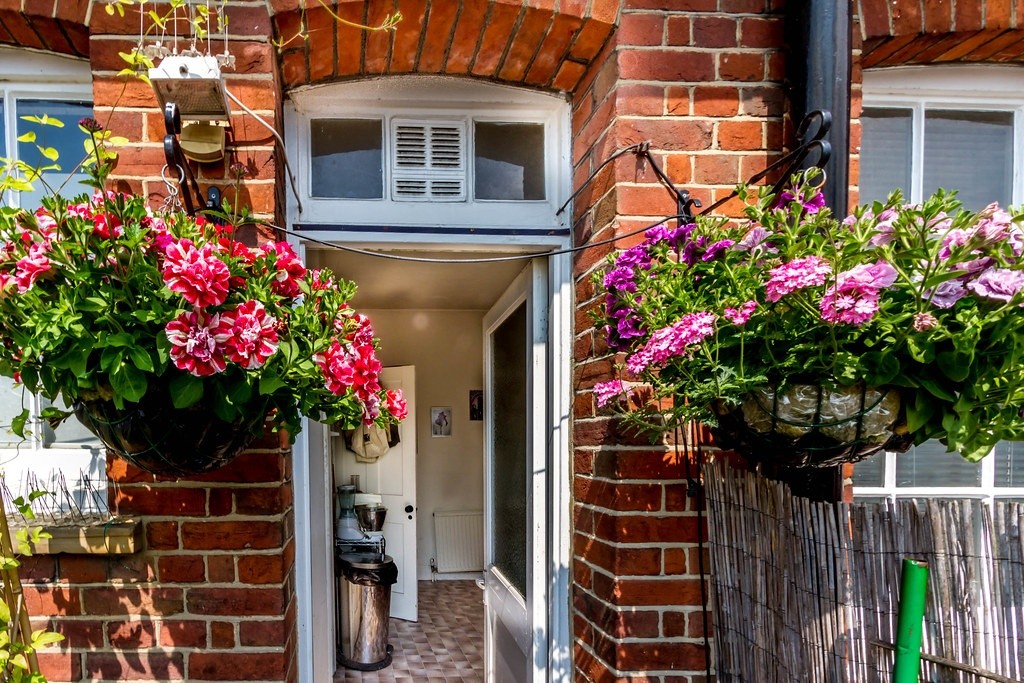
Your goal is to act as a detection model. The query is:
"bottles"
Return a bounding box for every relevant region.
[349,474,362,493]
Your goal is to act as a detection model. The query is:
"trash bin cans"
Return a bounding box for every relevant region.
[335,551,393,670]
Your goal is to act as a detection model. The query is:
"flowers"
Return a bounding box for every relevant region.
[5,200,409,429]
[606,192,1024,459]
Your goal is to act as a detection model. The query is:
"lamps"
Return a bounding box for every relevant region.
[149,55,232,122]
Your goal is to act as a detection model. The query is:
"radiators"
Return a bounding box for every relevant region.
[430,510,484,572]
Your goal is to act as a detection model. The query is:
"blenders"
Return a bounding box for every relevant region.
[336,484,366,542]
[339,492,388,542]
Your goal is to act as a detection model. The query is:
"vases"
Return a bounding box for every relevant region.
[728,366,912,474]
[68,371,277,473]
